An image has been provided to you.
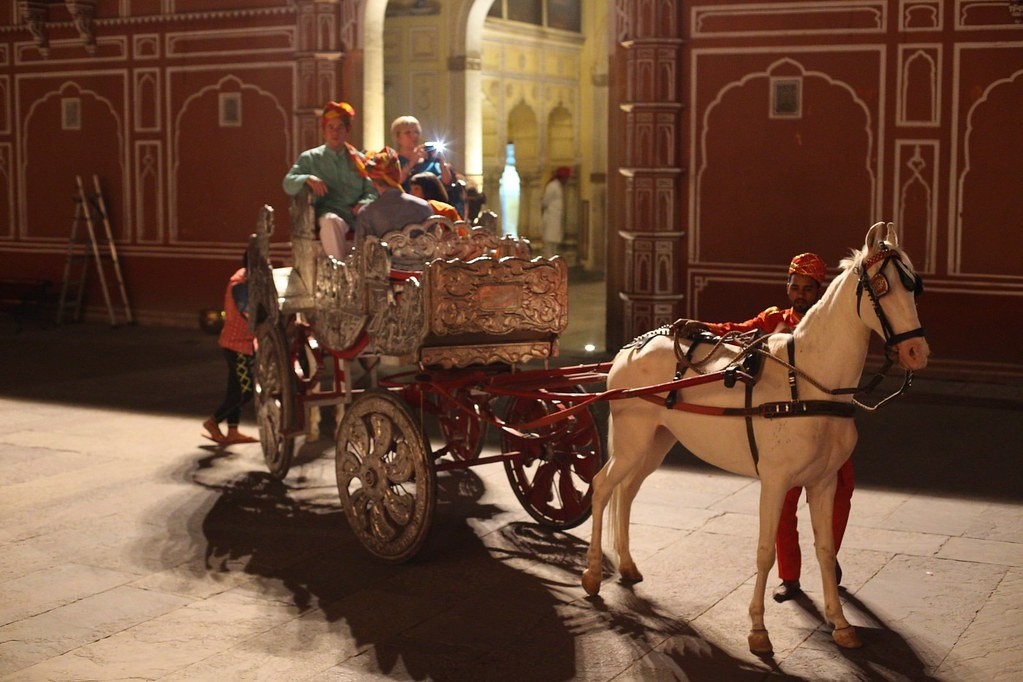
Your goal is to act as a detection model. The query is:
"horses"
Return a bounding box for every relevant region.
[580,221,931,656]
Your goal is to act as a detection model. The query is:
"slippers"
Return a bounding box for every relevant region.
[201,434,230,444]
[230,436,258,443]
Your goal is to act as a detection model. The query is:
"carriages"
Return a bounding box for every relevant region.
[246,190,930,659]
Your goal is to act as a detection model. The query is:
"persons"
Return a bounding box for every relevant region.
[540,165,572,260]
[390,116,467,219]
[282,101,379,261]
[667,254,856,598]
[345,142,437,248]
[203,247,261,444]
[406,170,469,238]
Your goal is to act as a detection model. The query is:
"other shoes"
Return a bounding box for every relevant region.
[835,559,842,585]
[773,580,800,602]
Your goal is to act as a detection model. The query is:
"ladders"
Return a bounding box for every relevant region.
[55,175,137,329]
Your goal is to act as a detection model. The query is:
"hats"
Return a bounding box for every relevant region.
[789,253,825,287]
[365,146,406,187]
[322,100,357,130]
[553,166,571,177]
